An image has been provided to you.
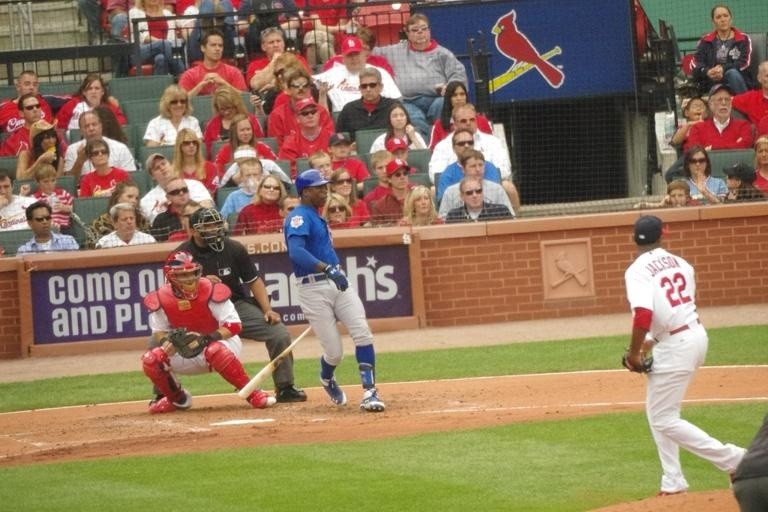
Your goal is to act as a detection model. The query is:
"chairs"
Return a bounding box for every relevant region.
[1,1,509,259]
[666,32,767,186]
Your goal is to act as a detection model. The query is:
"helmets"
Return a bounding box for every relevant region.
[189,207,225,252]
[295,168,328,198]
[163,250,202,301]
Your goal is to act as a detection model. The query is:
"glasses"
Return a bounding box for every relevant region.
[166,186,189,196]
[688,157,705,163]
[291,82,309,88]
[261,182,282,192]
[328,205,346,214]
[273,68,285,77]
[360,82,378,90]
[335,177,352,185]
[459,118,476,123]
[33,215,51,223]
[90,148,108,159]
[299,109,317,117]
[25,103,41,111]
[465,188,482,196]
[455,140,473,147]
[392,170,408,178]
[182,140,199,146]
[169,98,187,105]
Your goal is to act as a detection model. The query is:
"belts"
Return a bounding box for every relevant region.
[669,318,699,335]
[302,275,325,283]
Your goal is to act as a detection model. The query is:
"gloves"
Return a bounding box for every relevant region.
[326,266,348,291]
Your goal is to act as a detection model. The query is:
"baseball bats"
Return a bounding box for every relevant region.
[239,323,315,399]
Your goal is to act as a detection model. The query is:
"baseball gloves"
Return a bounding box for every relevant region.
[168,328,208,358]
[624,352,653,373]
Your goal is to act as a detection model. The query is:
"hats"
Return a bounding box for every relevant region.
[328,132,352,145]
[29,118,58,149]
[721,162,756,182]
[340,36,361,54]
[708,83,732,96]
[632,216,668,244]
[385,136,407,153]
[385,158,410,177]
[146,154,165,176]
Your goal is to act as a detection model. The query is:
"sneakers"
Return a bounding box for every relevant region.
[359,391,384,412]
[276,387,306,402]
[319,375,346,406]
[149,394,163,405]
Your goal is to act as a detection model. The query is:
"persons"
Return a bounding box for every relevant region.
[141,250,277,415]
[284,168,387,413]
[620,214,749,497]
[632,4,768,211]
[152,207,307,401]
